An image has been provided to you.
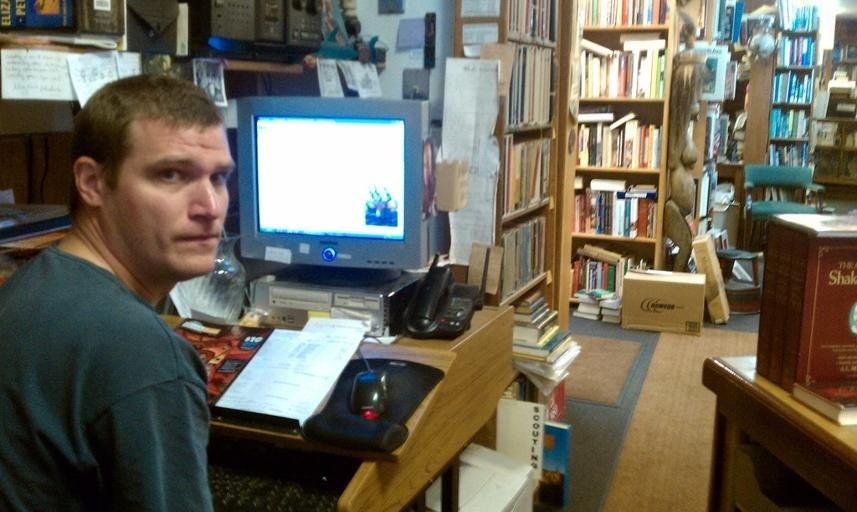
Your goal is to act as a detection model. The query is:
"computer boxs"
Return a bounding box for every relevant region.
[425,441,533,512]
[250,271,431,337]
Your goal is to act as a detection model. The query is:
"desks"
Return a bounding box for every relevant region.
[152,297,518,510]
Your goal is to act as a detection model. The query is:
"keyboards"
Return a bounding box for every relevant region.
[207,455,342,512]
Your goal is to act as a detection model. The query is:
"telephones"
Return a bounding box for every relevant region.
[402,254,478,340]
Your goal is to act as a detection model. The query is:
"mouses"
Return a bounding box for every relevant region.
[349,369,389,419]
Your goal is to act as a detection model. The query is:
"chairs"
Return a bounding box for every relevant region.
[724,162,825,286]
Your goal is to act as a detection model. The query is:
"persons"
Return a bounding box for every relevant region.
[1,75,237,510]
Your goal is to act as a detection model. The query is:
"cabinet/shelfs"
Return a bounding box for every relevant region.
[712,0,857,250]
[570,0,712,304]
[3,0,384,135]
[455,1,581,338]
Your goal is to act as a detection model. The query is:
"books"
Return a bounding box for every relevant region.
[687,0,819,250]
[174,318,275,406]
[816,40,856,147]
[570,0,670,323]
[502,1,580,397]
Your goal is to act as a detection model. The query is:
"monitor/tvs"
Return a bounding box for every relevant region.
[236,96,429,288]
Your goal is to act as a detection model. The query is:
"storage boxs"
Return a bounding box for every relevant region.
[690,233,732,325]
[620,265,707,336]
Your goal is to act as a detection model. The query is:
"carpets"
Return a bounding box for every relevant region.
[552,305,661,511]
